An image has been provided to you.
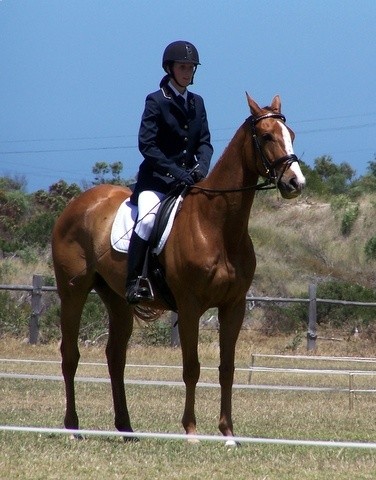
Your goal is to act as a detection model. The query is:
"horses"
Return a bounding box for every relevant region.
[51,90,308,451]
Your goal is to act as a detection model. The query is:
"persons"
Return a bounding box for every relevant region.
[123,40,214,305]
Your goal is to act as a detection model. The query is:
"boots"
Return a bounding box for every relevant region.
[126,231,150,305]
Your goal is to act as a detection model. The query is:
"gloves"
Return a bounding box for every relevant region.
[170,166,193,187]
[187,168,201,182]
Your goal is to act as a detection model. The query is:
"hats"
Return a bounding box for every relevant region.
[162,41,201,68]
